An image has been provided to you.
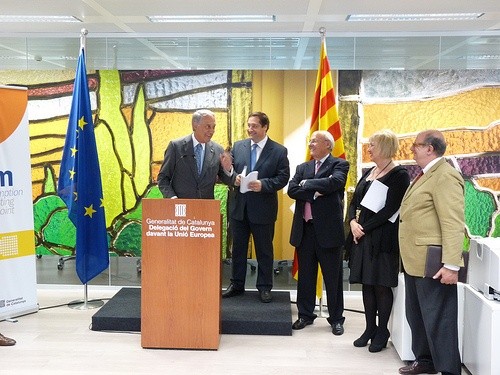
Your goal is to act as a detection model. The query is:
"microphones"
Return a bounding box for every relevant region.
[168,153,189,198]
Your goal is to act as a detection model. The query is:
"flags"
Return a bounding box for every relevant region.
[58,45,109,284]
[291,35,346,301]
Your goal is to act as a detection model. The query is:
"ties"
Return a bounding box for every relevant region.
[250,144,258,172]
[194,143,203,175]
[407,170,424,193]
[303,160,322,223]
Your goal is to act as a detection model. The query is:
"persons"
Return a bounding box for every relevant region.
[156,110,236,201]
[399,130,466,375]
[287,131,350,335]
[345,130,410,352]
[222,112,290,301]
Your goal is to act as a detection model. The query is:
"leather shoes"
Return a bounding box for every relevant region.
[0,333,16,346]
[259,288,273,302]
[222,282,245,298]
[331,322,345,336]
[399,360,438,375]
[293,318,313,330]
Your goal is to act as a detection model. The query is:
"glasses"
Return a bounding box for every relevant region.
[412,142,433,148]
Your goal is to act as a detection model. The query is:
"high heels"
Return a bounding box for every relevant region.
[353,325,378,347]
[368,328,390,353]
